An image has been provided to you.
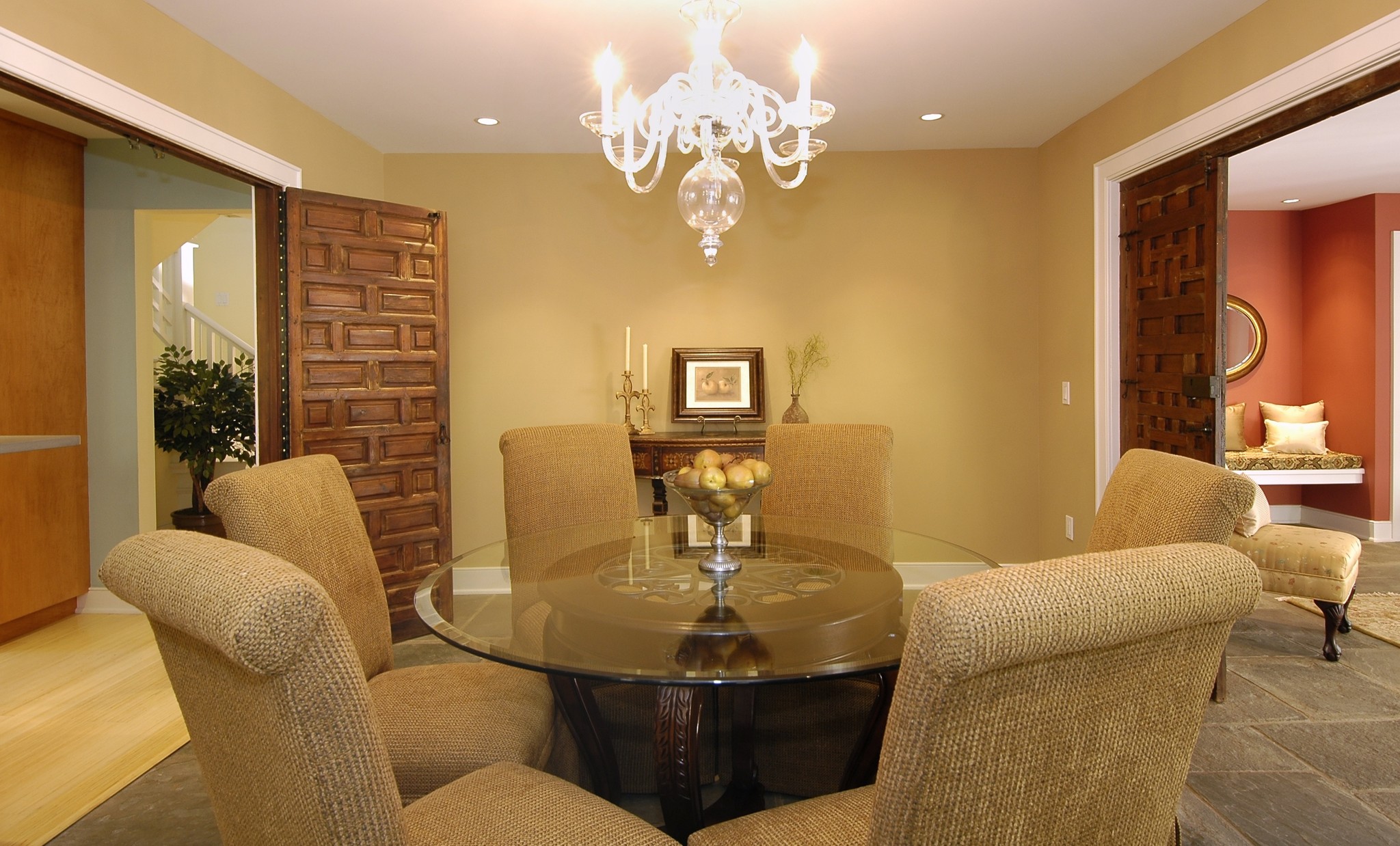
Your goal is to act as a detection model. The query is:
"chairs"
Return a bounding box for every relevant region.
[99,528,681,846]
[688,542,1264,846]
[1089,447,1259,703]
[205,454,561,805]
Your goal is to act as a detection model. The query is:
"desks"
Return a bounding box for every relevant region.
[413,513,1002,846]
[629,431,766,533]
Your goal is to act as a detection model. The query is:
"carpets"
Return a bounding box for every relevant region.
[1274,591,1400,649]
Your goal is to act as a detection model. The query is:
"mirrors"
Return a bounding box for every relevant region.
[1227,294,1267,384]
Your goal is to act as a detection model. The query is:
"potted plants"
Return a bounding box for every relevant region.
[153,344,255,540]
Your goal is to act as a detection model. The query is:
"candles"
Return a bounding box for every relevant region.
[642,343,648,389]
[625,326,631,371]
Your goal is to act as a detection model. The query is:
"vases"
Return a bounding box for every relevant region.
[782,395,808,424]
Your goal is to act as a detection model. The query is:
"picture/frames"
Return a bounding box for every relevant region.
[670,346,766,424]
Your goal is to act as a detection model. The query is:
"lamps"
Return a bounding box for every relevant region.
[578,0,835,267]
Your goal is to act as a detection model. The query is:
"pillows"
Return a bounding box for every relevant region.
[1234,469,1272,539]
[1225,402,1246,451]
[1263,419,1329,454]
[1260,399,1325,450]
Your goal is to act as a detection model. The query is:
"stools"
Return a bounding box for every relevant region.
[1226,523,1362,662]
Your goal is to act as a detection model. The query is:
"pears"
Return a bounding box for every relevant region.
[674,449,772,522]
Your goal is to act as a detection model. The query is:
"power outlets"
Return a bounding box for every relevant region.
[1065,514,1073,542]
